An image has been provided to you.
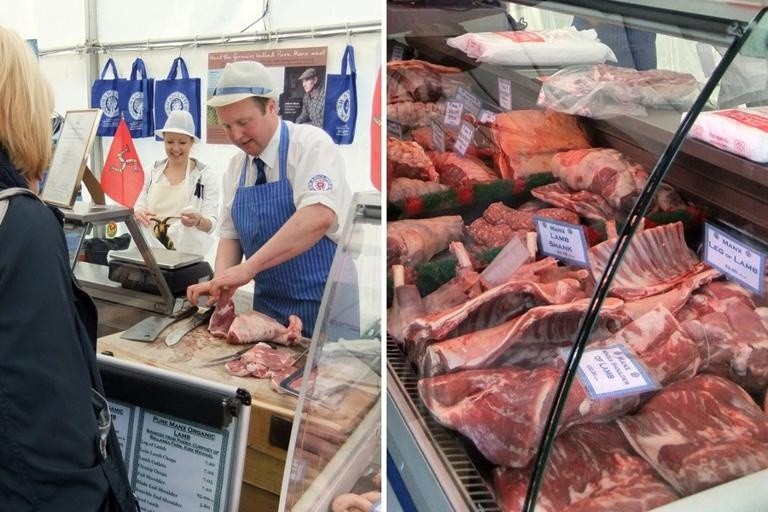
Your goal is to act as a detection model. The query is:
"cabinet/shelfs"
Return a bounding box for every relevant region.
[388,0,764,510]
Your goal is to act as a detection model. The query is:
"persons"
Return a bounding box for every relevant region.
[131,109,220,262]
[294,69,326,131]
[0,23,141,512]
[187,61,360,345]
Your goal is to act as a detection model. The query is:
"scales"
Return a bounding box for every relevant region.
[108,248,214,297]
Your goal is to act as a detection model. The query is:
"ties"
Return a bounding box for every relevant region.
[253,158,266,185]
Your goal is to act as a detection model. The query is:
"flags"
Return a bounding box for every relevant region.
[101,120,145,208]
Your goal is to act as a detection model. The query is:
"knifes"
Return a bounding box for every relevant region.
[119,304,198,344]
[164,307,216,346]
[198,347,247,368]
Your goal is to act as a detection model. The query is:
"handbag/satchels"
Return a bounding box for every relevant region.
[323,46,357,144]
[91,58,201,140]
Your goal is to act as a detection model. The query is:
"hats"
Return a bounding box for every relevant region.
[298,68,316,80]
[206,61,274,106]
[155,110,200,144]
